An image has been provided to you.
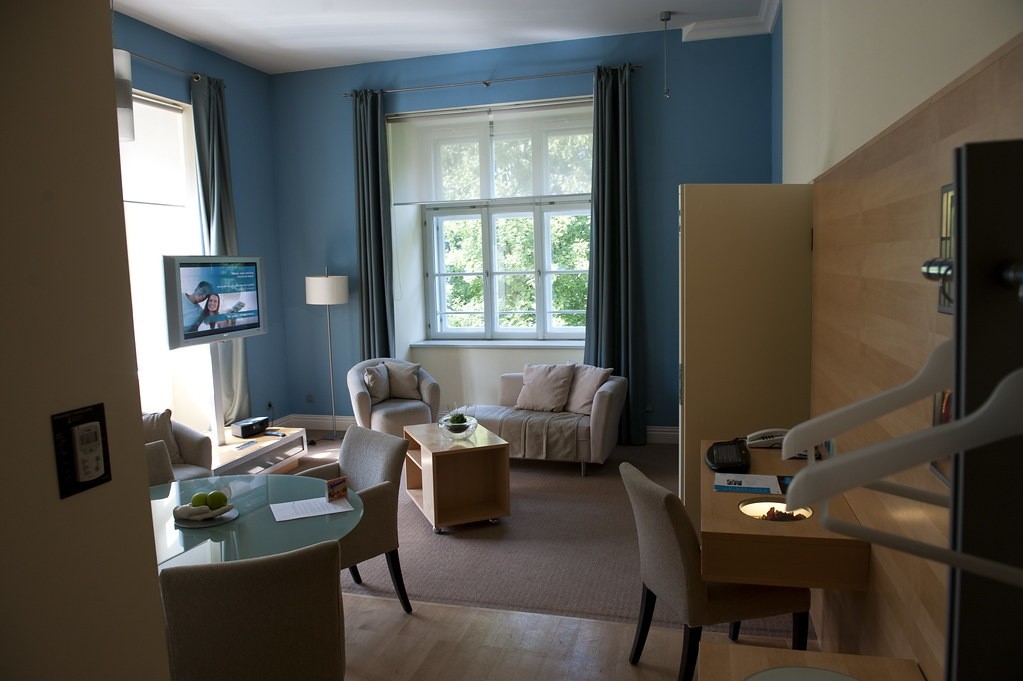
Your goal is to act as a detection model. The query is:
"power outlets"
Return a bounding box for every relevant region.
[266,399,272,410]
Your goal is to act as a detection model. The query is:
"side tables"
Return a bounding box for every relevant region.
[398,419,510,533]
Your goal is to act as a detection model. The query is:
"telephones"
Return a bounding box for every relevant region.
[747,428,790,447]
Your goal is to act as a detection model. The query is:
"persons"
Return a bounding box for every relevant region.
[196,294,237,331]
[181,281,220,333]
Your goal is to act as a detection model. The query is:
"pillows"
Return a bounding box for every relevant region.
[385,361,422,400]
[565,360,614,413]
[514,362,576,412]
[364,360,390,407]
[141,407,184,463]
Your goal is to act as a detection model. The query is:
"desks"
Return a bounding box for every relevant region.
[146,473,364,576]
[698,439,869,592]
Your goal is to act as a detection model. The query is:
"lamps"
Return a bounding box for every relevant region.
[305,264,350,439]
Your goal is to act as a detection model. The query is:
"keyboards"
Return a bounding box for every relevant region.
[706,440,751,474]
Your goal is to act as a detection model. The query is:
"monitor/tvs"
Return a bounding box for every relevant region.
[163,256,267,350]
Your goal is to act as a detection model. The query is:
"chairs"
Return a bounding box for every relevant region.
[618,462,811,680]
[286,425,412,615]
[162,540,347,681]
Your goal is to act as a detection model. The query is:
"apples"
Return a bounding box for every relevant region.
[207,491,227,510]
[192,492,207,507]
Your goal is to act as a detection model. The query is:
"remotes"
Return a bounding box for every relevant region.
[265,431,285,437]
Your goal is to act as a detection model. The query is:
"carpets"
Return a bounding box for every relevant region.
[304,429,818,640]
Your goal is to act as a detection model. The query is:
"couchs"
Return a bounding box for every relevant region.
[143,413,213,487]
[346,357,439,438]
[440,372,628,474]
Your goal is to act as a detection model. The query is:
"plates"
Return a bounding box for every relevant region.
[173,503,239,528]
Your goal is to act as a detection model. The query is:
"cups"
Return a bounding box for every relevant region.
[447,401,476,417]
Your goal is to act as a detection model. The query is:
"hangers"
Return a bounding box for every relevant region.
[781,333,957,508]
[785,367,1023,591]
